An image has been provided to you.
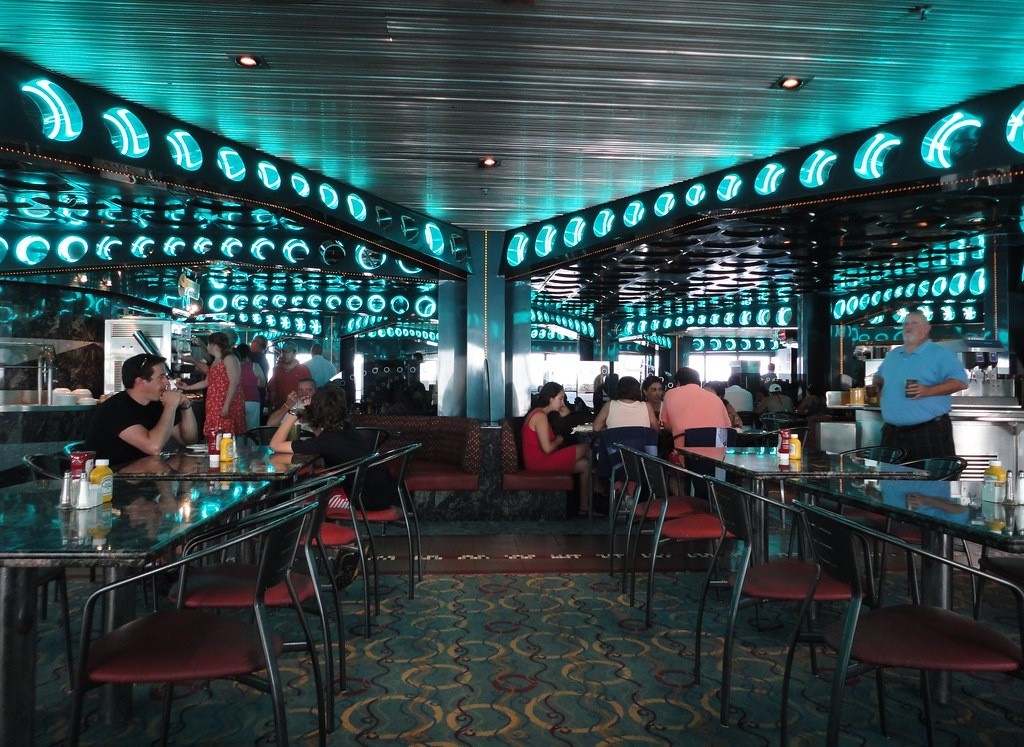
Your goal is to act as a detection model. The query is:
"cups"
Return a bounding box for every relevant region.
[826,391,841,406]
[906,380,917,398]
[289,425,301,441]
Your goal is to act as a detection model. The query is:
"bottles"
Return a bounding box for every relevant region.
[1017,470,1024,505]
[61,471,72,509]
[76,471,90,509]
[1005,470,1014,500]
[210,427,237,462]
[867,386,879,406]
[68,452,113,502]
[983,461,1006,486]
[778,429,801,459]
[841,387,867,406]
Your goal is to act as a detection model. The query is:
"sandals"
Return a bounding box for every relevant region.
[593,487,610,498]
[577,509,605,517]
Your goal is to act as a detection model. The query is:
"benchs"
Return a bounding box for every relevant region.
[499,415,575,492]
[351,414,481,492]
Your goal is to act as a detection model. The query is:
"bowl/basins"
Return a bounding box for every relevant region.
[52,388,97,406]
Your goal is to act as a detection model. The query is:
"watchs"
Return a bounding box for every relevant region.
[289,407,302,419]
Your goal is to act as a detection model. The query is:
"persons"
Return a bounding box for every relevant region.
[266,377,322,440]
[520,382,605,518]
[761,363,777,380]
[271,382,392,589]
[83,354,198,575]
[871,310,969,481]
[175,332,336,444]
[593,367,822,512]
[112,453,198,549]
[594,364,608,389]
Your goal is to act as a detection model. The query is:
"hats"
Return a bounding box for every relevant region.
[768,384,781,392]
[281,341,296,353]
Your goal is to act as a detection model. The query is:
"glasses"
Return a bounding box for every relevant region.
[140,353,153,368]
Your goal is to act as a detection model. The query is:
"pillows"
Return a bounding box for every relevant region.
[403,432,430,458]
[433,430,465,465]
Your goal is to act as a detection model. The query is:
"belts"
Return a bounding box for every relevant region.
[884,413,947,428]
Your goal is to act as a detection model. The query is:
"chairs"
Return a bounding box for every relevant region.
[22,425,422,747]
[588,410,1024,747]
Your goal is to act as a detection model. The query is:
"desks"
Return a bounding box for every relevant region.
[0,441,76,474]
[786,481,1024,704]
[674,447,931,602]
[0,479,271,747]
[119,450,323,563]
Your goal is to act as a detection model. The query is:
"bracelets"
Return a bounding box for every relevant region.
[179,398,192,410]
[284,403,291,410]
